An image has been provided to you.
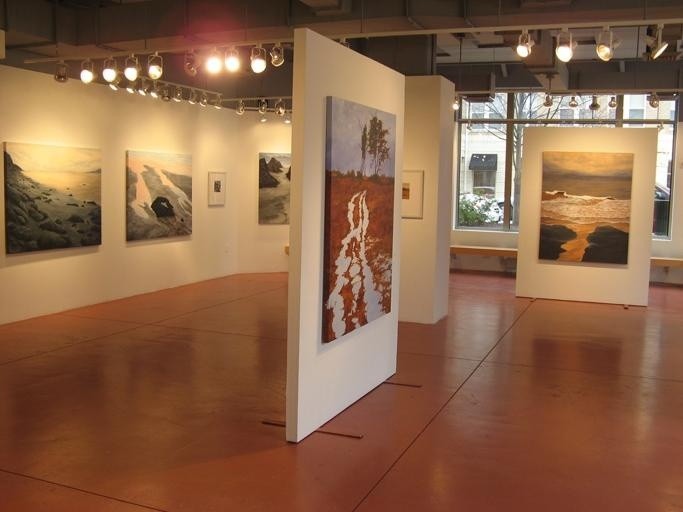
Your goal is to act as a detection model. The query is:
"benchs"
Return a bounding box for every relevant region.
[447,244,516,272]
[648,255,682,276]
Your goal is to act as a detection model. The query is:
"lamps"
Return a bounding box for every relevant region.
[447,18,677,111]
[47,33,293,131]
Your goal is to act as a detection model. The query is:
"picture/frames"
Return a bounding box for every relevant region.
[398,168,425,219]
[206,170,227,207]
